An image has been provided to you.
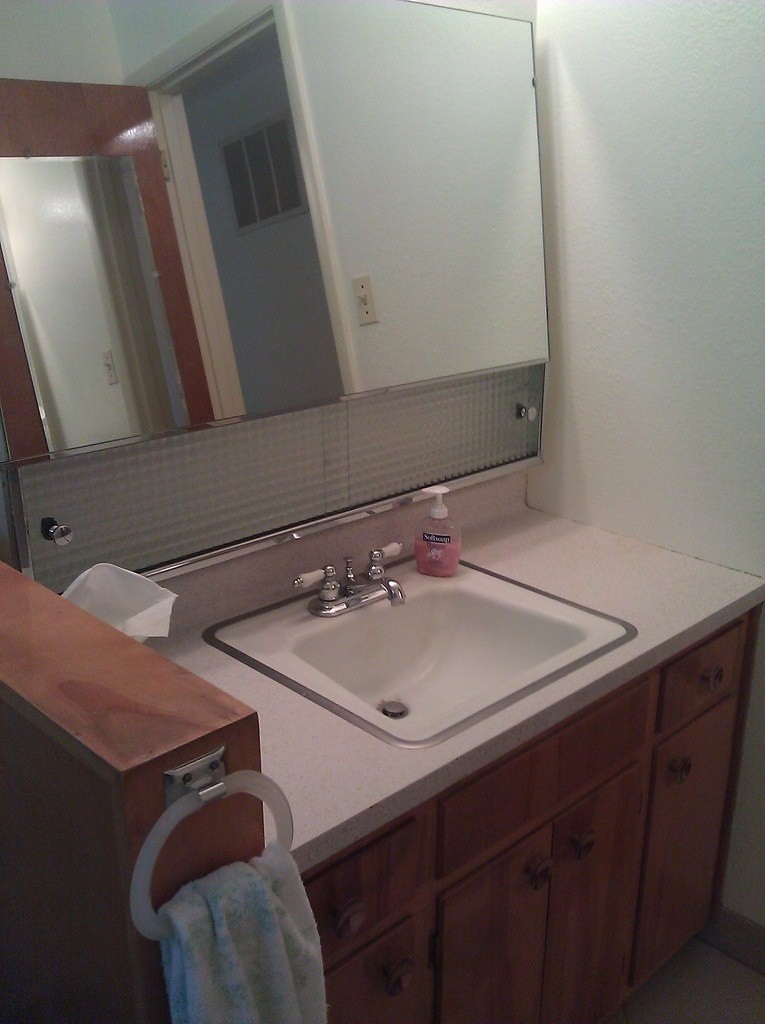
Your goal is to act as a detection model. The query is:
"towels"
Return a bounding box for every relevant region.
[155,842,329,1024]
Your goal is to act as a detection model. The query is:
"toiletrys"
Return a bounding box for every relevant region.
[414,484,463,577]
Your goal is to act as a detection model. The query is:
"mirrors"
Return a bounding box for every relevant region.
[0,0,551,467]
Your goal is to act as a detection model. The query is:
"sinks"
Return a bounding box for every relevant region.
[211,582,627,744]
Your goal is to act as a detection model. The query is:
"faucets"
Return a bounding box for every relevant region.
[341,556,407,607]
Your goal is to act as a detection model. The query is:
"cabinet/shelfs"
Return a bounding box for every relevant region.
[299,604,759,1024]
